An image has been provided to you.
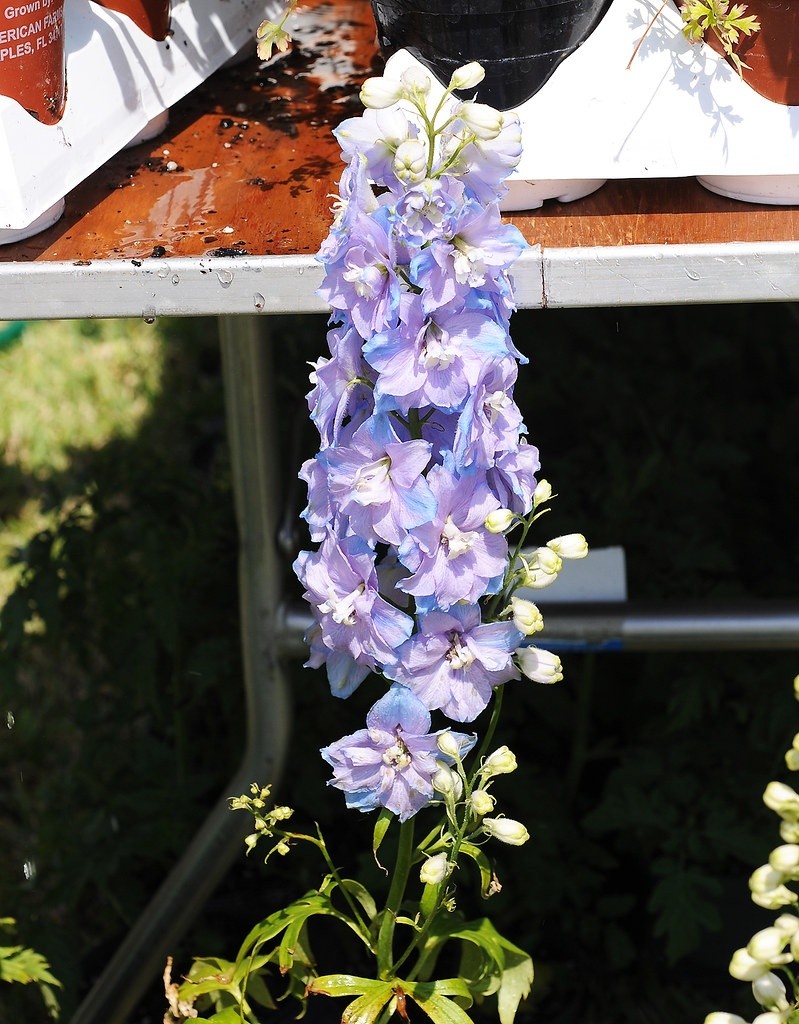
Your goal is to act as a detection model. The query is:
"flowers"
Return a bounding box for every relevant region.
[162,62,799,1024]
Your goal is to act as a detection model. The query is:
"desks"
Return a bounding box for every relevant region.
[0,0,799,1024]
